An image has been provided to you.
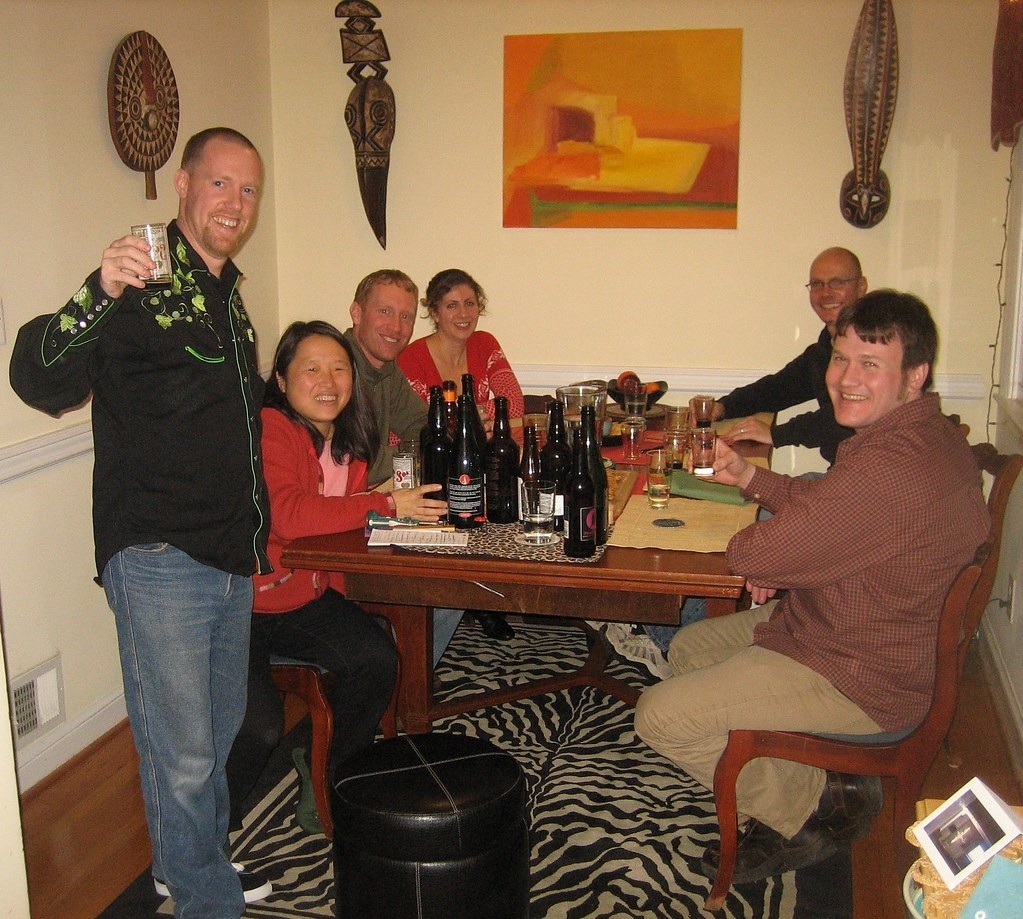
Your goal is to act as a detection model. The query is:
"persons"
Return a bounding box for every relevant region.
[227,320,449,835]
[636,288,994,886]
[385,269,524,446]
[689,247,870,522]
[10,129,272,919]
[342,270,432,492]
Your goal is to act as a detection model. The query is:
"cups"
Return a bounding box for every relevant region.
[622,420,643,460]
[689,428,717,477]
[663,431,687,472]
[648,449,672,508]
[624,382,646,422]
[521,480,556,544]
[693,395,715,427]
[392,440,421,491]
[665,406,691,447]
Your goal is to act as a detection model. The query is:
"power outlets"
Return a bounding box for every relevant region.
[1006,573,1016,623]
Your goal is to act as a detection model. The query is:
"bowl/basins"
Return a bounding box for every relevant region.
[607,380,668,412]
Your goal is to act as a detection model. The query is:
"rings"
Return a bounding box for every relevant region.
[740,428,744,433]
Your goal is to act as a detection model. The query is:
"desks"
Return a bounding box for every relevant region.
[280,406,779,739]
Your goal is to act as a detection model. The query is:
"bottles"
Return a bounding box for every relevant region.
[419,375,609,559]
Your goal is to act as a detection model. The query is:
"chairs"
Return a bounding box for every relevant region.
[706,414,1023,908]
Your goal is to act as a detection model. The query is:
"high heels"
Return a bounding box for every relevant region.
[463,609,515,640]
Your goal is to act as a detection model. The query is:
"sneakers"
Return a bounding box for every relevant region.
[701,817,839,884]
[809,770,883,840]
[585,620,675,680]
[153,863,273,903]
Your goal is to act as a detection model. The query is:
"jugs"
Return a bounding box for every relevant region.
[556,380,606,452]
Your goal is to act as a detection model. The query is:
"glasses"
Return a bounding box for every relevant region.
[806,278,859,292]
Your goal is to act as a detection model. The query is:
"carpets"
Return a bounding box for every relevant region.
[93,608,857,919]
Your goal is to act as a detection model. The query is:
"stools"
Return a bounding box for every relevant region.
[326,734,532,919]
[261,657,403,838]
